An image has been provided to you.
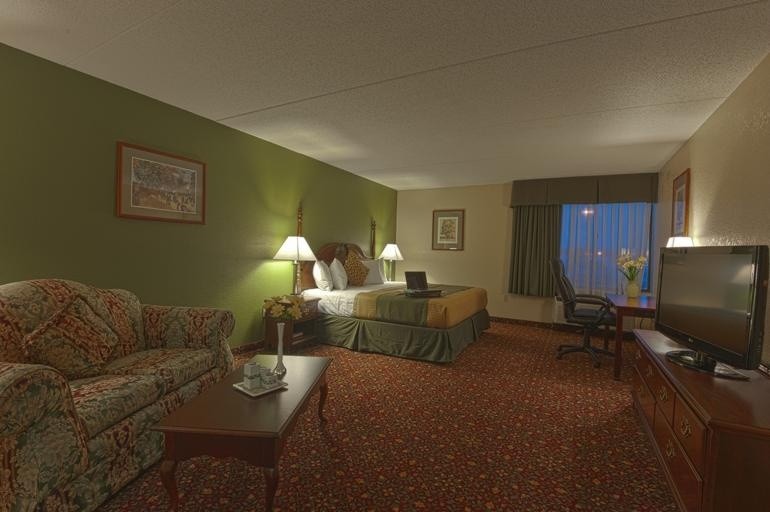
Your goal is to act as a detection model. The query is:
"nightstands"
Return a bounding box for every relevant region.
[264,295,323,355]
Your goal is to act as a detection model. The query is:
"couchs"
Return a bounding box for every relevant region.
[1,278,236,512]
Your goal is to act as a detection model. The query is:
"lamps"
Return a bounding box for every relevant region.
[273,236,317,295]
[665,236,694,248]
[377,243,404,281]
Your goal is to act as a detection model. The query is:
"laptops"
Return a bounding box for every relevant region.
[403,271,442,298]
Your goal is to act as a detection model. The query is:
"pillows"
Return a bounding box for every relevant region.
[380,258,385,283]
[360,259,384,284]
[344,254,369,287]
[313,261,333,292]
[328,258,348,290]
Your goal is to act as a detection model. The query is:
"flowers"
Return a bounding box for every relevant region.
[617,254,648,281]
[263,295,310,323]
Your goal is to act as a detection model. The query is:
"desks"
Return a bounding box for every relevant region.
[604,293,656,381]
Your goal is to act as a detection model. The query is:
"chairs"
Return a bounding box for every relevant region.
[550,257,617,368]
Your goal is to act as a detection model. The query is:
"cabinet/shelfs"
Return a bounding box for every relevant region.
[630,329,770,512]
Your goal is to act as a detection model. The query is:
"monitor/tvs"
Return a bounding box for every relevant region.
[653,244,768,381]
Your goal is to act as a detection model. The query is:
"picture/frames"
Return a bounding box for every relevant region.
[672,168,691,236]
[115,141,207,225]
[432,210,464,250]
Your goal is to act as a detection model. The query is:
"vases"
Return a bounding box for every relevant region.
[626,281,640,297]
[274,323,287,375]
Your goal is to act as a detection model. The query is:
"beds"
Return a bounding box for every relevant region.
[298,207,491,364]
